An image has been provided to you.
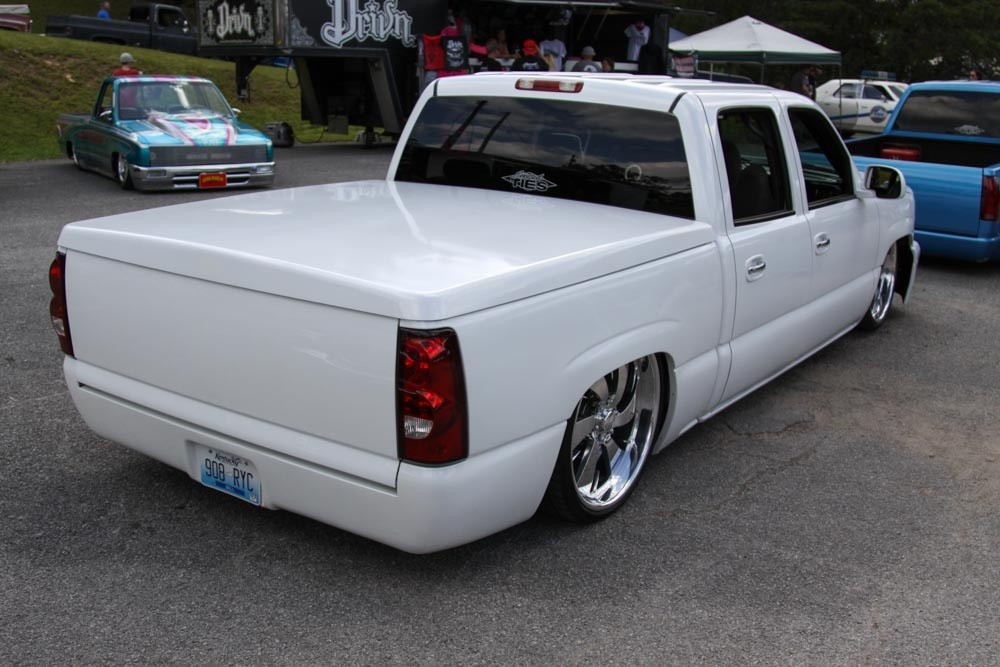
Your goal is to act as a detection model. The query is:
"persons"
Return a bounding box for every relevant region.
[790,63,823,98]
[970,68,983,80]
[96,0,112,20]
[467,29,566,72]
[111,52,143,107]
[571,46,616,73]
[624,16,650,63]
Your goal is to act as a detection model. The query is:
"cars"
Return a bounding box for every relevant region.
[813,78,912,133]
[58,73,277,193]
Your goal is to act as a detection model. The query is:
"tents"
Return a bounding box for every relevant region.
[668,15,841,135]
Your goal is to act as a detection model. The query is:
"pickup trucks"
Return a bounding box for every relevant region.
[43,0,215,57]
[796,78,1000,275]
[47,68,922,558]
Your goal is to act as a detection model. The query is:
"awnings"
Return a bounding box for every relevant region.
[509,1,716,40]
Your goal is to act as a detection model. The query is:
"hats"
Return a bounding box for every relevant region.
[523,40,537,54]
[582,47,595,55]
[120,53,137,62]
